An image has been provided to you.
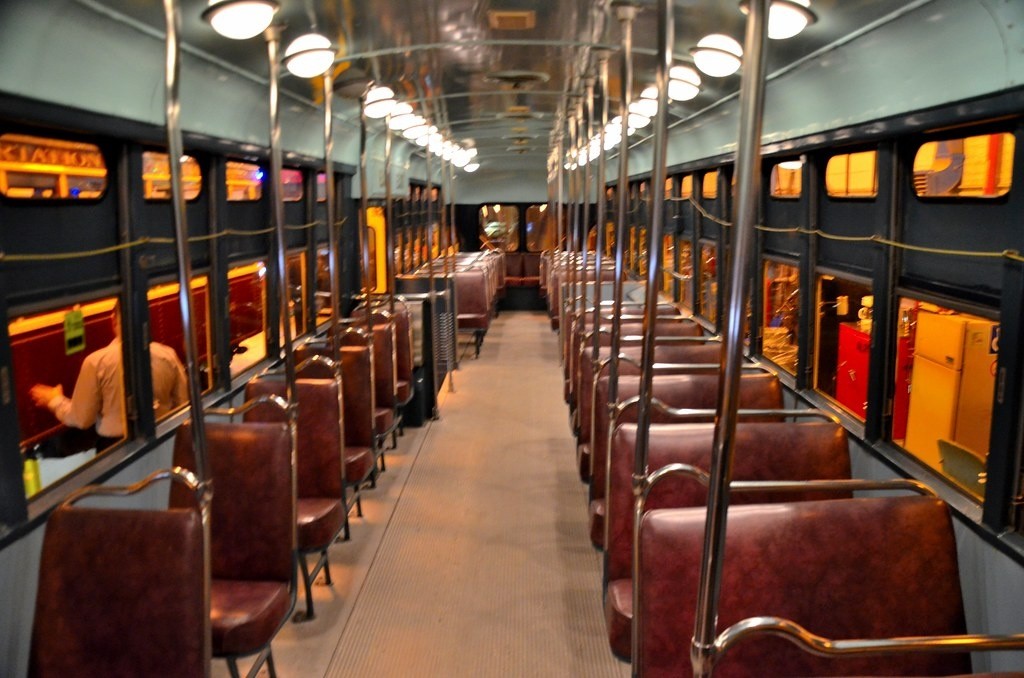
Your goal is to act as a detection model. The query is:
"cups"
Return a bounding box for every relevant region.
[858,295,873,330]
[836,295,848,315]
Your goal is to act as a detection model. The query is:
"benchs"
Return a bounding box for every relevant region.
[416,251,975,678]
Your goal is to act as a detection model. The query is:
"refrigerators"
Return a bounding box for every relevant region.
[905,312,1000,470]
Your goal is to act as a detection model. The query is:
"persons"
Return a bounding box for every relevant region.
[30,304,188,458]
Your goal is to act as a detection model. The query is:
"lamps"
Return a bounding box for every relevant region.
[201,0,280,40]
[628,78,704,128]
[279,47,339,78]
[365,98,425,139]
[739,0,818,40]
[688,47,743,78]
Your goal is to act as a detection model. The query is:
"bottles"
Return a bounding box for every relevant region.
[21,443,43,501]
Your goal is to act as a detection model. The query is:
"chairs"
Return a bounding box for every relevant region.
[25,295,414,678]
